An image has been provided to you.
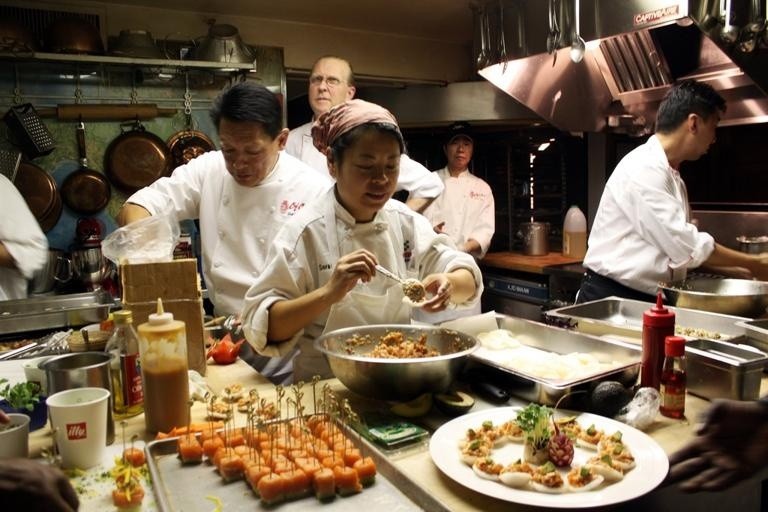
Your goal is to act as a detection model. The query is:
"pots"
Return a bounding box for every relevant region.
[9,117,218,294]
[51,18,256,75]
[520,222,550,257]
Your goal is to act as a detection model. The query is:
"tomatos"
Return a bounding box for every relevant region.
[207,332,245,362]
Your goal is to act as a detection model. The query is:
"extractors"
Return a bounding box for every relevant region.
[468,0,767,137]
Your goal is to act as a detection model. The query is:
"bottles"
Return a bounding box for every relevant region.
[639,290,676,392]
[137,295,190,436]
[103,309,146,420]
[562,205,587,259]
[659,336,688,419]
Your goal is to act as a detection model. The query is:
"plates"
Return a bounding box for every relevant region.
[429,405,669,510]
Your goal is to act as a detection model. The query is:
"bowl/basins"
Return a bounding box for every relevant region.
[655,276,768,316]
[317,316,482,401]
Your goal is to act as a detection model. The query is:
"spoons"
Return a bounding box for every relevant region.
[374,265,426,299]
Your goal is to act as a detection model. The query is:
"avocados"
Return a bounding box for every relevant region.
[434,388,475,412]
[389,392,431,417]
[591,381,629,412]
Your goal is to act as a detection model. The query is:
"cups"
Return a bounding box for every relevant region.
[0,414,32,464]
[20,330,115,473]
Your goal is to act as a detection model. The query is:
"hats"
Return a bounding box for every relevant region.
[444,121,475,145]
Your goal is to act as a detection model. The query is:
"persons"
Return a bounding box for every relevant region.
[668,384,768,494]
[111,82,334,385]
[242,99,486,383]
[0,172,49,301]
[569,78,768,309]
[282,54,445,213]
[410,127,496,325]
